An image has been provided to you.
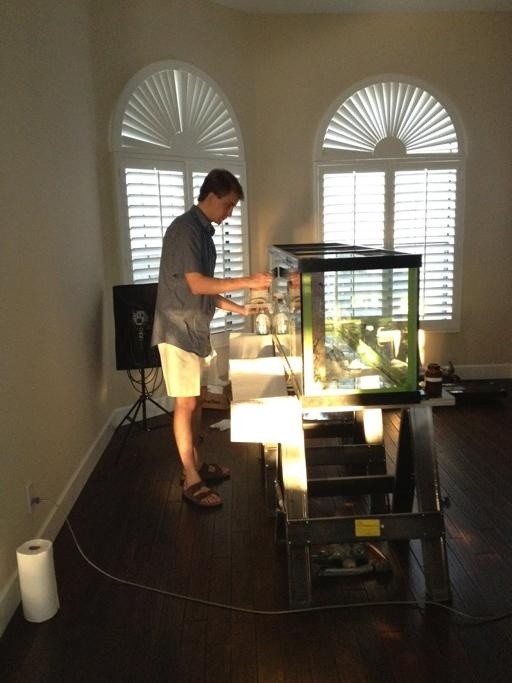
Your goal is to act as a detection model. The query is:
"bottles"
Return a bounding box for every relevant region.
[255,309,288,335]
[425,363,442,398]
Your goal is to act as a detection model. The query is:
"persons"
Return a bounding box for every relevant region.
[148,166,272,509]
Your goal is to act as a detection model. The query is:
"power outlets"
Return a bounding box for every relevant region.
[25,481,36,515]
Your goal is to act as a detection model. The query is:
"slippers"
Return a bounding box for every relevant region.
[197,463,231,483]
[182,482,223,508]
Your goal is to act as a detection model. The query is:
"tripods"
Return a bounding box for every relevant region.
[108,369,205,467]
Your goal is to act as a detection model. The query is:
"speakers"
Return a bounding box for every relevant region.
[111,282,163,370]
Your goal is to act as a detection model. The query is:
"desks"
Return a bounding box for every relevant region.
[226,356,456,613]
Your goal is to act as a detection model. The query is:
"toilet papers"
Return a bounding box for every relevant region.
[16,539,62,623]
